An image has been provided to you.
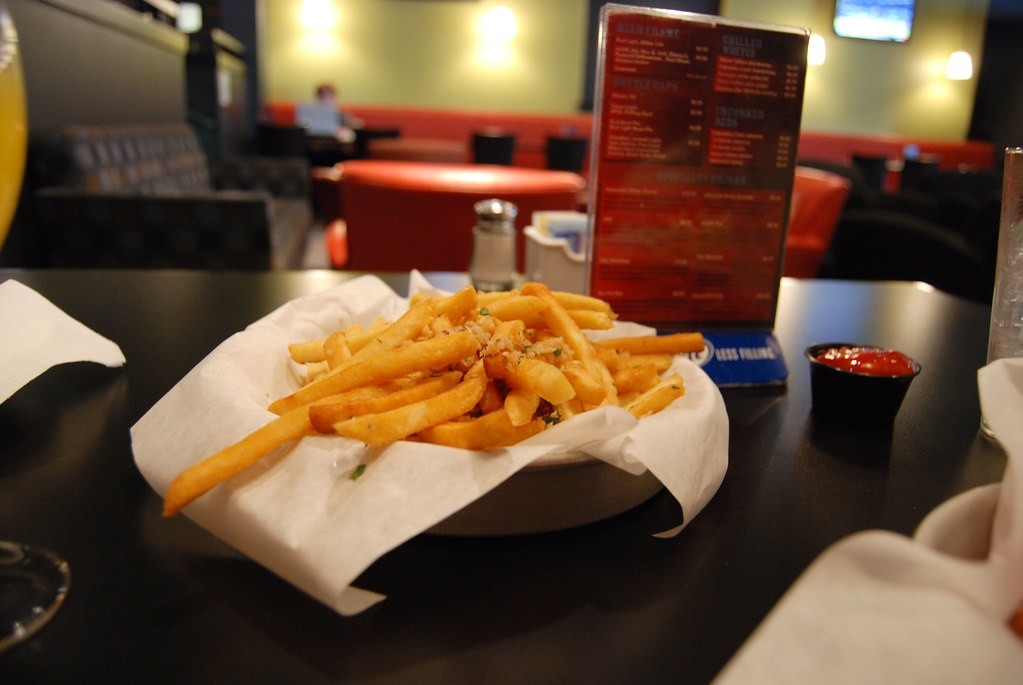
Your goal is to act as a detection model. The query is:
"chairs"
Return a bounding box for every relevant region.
[780,167,852,271]
[335,159,585,268]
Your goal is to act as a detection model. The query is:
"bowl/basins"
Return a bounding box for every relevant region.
[805,342,922,428]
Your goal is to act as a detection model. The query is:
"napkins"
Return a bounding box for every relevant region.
[704,356,1023,685]
[0,274,129,406]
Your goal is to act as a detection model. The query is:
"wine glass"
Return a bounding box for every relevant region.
[0,8,70,652]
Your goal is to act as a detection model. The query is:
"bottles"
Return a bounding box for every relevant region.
[471,199,518,291]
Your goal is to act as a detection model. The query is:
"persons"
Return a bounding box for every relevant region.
[298,85,365,143]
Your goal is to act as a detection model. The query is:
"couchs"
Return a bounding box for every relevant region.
[271,100,594,169]
[796,152,1003,301]
[34,126,316,274]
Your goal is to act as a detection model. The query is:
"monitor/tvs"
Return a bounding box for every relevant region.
[832,0,917,43]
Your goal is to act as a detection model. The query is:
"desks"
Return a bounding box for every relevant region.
[0,262,1023,685]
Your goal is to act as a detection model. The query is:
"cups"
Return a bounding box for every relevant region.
[979,147,1023,443]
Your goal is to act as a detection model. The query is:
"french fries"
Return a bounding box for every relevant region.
[161,282,708,519]
